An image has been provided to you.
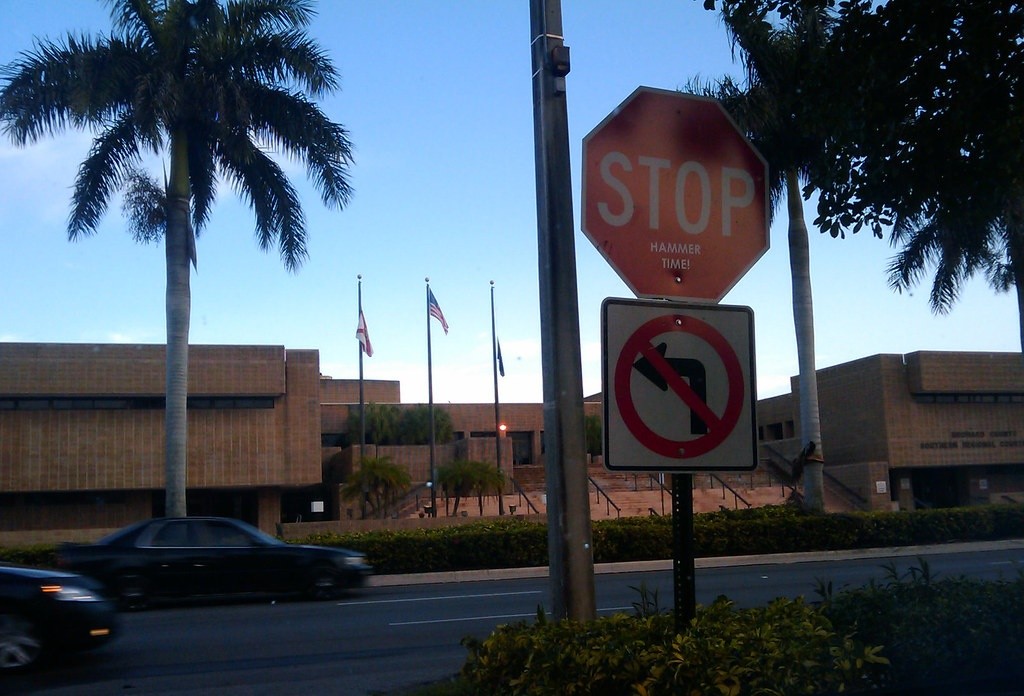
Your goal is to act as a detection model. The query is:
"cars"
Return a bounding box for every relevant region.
[0,565,119,674]
[51,515,372,610]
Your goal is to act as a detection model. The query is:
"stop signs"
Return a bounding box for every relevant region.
[579,84,771,305]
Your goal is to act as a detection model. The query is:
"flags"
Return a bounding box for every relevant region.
[429,289,449,335]
[355,309,374,357]
[496,341,505,377]
